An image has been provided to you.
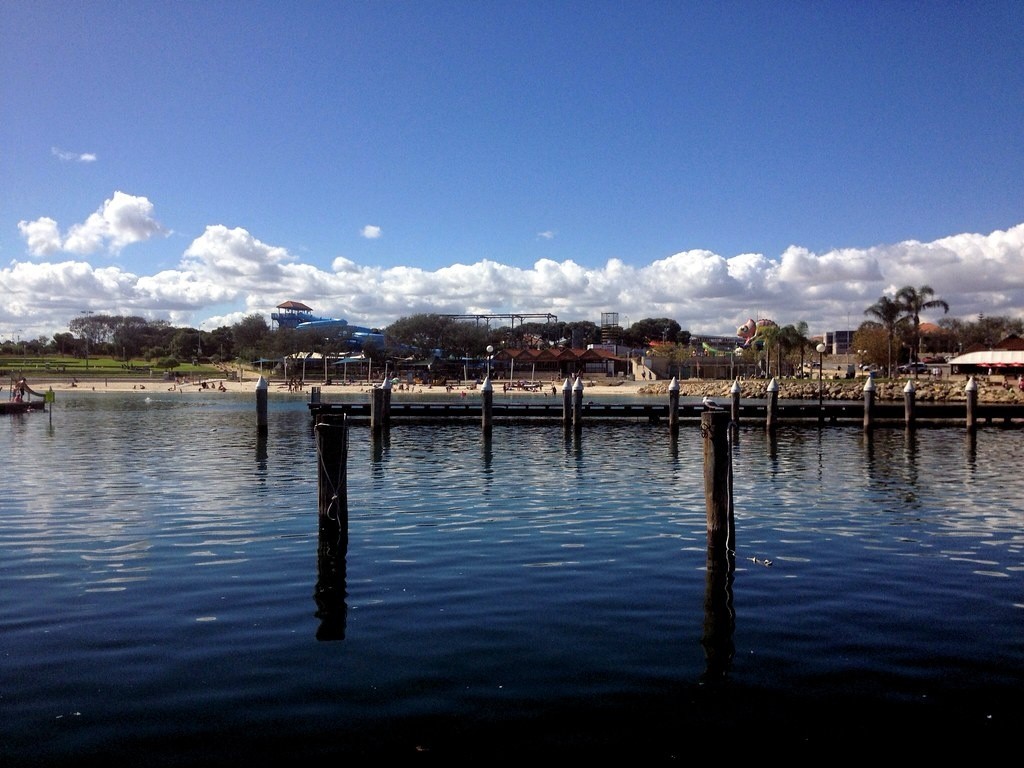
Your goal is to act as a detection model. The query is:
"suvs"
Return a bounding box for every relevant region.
[902,362,928,374]
[863,363,880,372]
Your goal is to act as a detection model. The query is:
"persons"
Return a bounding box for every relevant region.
[736,360,1024,398]
[642,370,653,382]
[66,361,312,399]
[320,365,588,401]
[0,377,34,414]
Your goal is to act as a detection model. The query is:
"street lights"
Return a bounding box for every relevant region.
[857,349,867,382]
[486,344,493,379]
[816,342,825,405]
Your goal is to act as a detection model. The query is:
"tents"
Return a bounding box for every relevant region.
[236,344,495,383]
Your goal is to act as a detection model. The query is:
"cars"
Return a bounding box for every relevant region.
[921,355,956,364]
[808,361,821,369]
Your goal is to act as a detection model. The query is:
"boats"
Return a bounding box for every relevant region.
[0,367,47,414]
